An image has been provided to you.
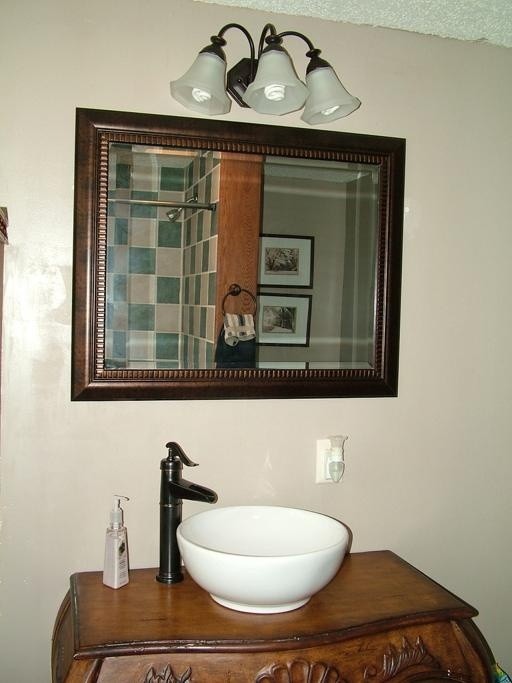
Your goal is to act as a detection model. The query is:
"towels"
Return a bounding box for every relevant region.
[214,326,256,369]
[224,313,256,347]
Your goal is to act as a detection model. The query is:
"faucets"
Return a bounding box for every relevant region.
[156,440,218,585]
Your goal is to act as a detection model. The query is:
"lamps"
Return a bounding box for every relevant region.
[170,23,361,125]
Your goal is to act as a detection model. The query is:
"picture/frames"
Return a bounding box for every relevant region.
[71,107,406,401]
[256,292,312,347]
[258,233,314,289]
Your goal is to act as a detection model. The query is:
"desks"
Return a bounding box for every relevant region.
[49,550,498,681]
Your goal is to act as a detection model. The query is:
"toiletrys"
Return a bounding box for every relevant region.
[103,495,132,588]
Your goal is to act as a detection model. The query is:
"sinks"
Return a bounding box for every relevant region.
[177,505,351,615]
